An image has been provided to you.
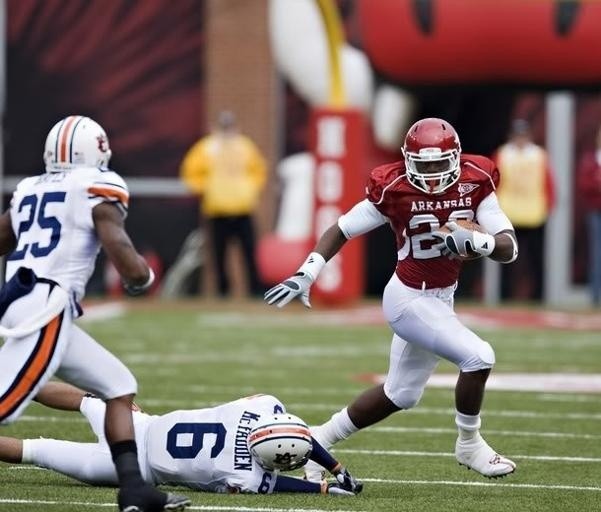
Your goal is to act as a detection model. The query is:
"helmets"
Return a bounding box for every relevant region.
[400,117,462,194]
[246,413,313,472]
[42,115,112,173]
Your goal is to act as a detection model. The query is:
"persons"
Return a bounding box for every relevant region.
[177,108,272,302]
[0,114,192,512]
[0,378,364,498]
[572,125,601,305]
[491,116,555,305]
[261,116,520,486]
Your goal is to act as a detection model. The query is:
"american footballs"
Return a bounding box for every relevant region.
[438,219,488,260]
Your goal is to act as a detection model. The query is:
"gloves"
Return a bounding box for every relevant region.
[326,466,363,495]
[432,221,495,261]
[263,252,327,308]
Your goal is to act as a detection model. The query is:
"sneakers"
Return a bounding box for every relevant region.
[455,435,517,480]
[118,486,191,512]
